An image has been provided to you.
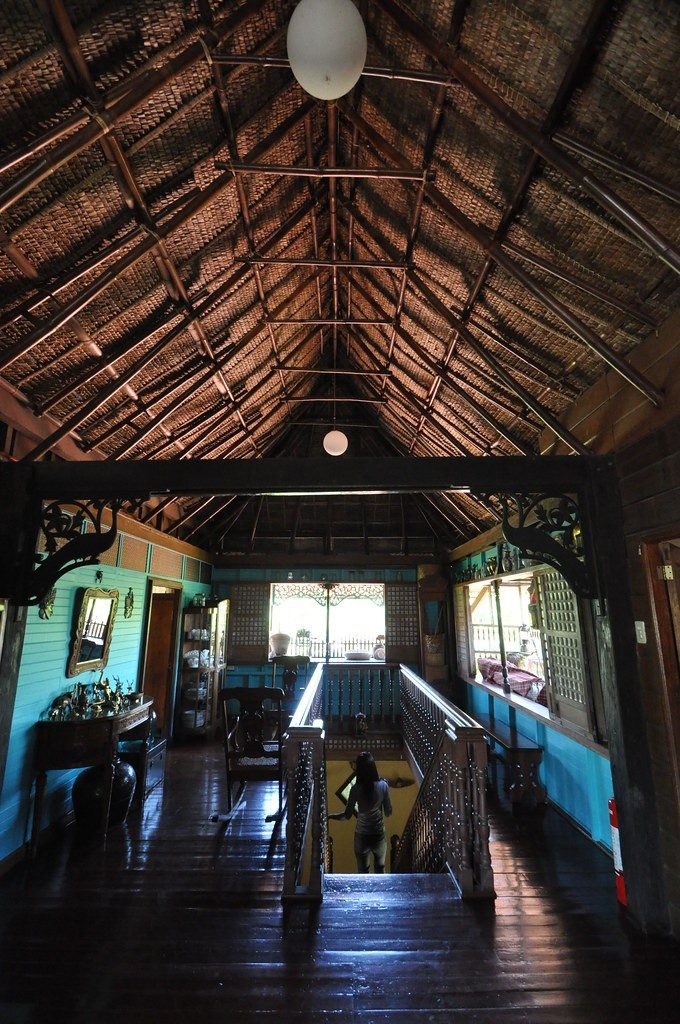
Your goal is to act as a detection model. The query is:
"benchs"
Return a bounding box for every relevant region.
[472,712,546,805]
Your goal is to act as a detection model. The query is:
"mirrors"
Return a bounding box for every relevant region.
[68,587,120,679]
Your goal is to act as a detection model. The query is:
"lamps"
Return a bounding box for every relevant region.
[287,0,367,101]
[322,429,350,457]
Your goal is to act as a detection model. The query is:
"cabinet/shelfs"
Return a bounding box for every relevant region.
[122,738,167,794]
[179,594,230,744]
[225,664,422,720]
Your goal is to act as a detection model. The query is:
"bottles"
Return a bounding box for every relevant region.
[475,566,480,579]
[481,556,497,577]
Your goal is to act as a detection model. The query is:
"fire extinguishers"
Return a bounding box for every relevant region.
[607,797,627,907]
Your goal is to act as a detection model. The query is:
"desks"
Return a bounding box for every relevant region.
[27,695,154,861]
[263,709,293,719]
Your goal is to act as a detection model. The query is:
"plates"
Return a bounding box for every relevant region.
[123,692,145,703]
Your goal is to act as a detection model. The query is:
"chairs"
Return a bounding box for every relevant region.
[265,657,310,740]
[212,685,289,823]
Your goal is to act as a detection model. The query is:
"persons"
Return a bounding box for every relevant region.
[328,751,393,873]
